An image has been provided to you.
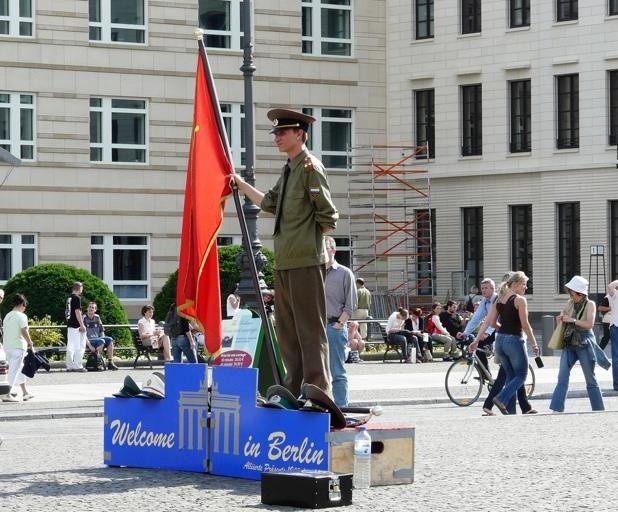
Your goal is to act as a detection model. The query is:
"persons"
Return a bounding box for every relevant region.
[351,277,371,338]
[347,321,365,361]
[426,302,458,361]
[226,285,241,320]
[549,275,604,412]
[263,294,276,327]
[474,302,482,333]
[490,271,539,415]
[3,293,35,402]
[608,280,618,390]
[457,278,499,380]
[137,304,170,360]
[325,235,359,413]
[0,289,4,344]
[421,306,430,316]
[439,300,468,340]
[386,307,427,363]
[404,308,435,363]
[228,109,339,403]
[171,300,198,363]
[468,270,537,416]
[464,284,478,316]
[82,302,119,370]
[64,282,87,372]
[597,296,612,350]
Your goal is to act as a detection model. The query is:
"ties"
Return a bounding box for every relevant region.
[272,165,291,236]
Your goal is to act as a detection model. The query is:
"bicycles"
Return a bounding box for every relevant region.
[446,336,537,408]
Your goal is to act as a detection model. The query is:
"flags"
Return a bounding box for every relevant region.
[176,42,233,363]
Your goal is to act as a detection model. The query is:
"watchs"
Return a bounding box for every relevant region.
[573,318,576,325]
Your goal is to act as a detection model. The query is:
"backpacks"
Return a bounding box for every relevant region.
[86,350,106,371]
[466,294,477,312]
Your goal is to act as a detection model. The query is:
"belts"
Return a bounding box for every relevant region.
[325,317,339,324]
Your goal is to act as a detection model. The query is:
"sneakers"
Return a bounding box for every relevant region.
[108,363,118,370]
[2,395,20,402]
[22,391,34,401]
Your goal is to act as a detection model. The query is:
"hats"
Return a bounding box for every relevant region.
[134,373,165,399]
[302,383,346,429]
[262,384,301,410]
[112,375,141,398]
[266,109,317,134]
[565,275,590,296]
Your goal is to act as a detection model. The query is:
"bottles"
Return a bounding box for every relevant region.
[534,351,543,369]
[353,427,371,489]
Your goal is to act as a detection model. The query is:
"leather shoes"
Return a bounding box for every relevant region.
[473,374,493,381]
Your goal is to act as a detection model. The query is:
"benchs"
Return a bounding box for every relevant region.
[378,322,460,363]
[130,326,162,370]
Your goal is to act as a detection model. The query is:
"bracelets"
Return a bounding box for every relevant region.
[532,346,536,347]
[336,319,344,326]
[27,342,33,346]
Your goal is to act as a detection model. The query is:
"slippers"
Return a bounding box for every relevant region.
[492,400,510,415]
[524,408,538,414]
[482,412,497,416]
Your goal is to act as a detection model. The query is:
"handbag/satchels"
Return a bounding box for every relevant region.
[423,350,433,361]
[347,349,360,363]
[405,343,417,363]
[547,320,568,350]
